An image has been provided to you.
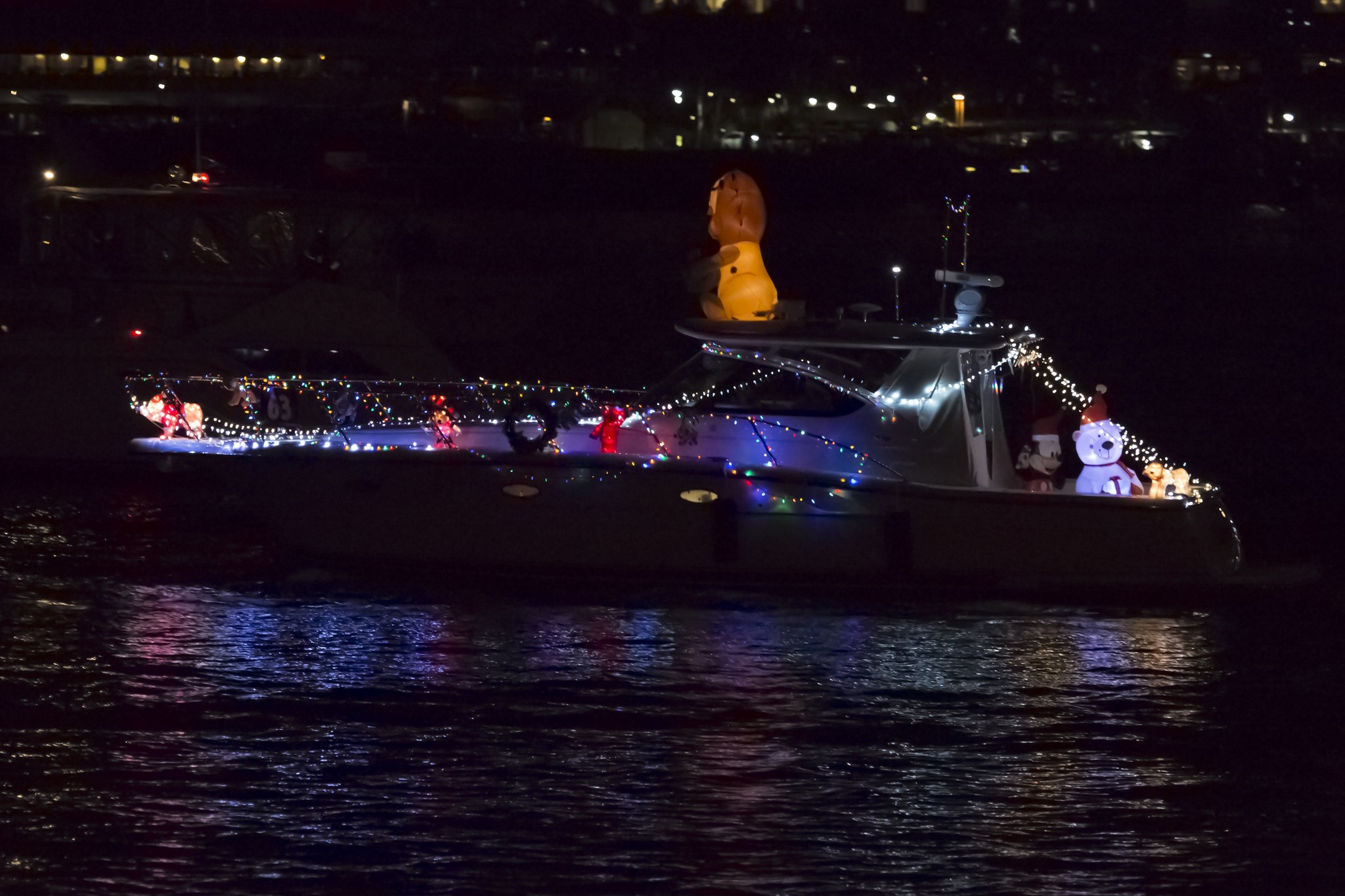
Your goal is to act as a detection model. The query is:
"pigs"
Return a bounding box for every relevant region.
[139,401,202,440]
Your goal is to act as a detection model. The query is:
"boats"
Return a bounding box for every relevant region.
[122,162,1330,597]
